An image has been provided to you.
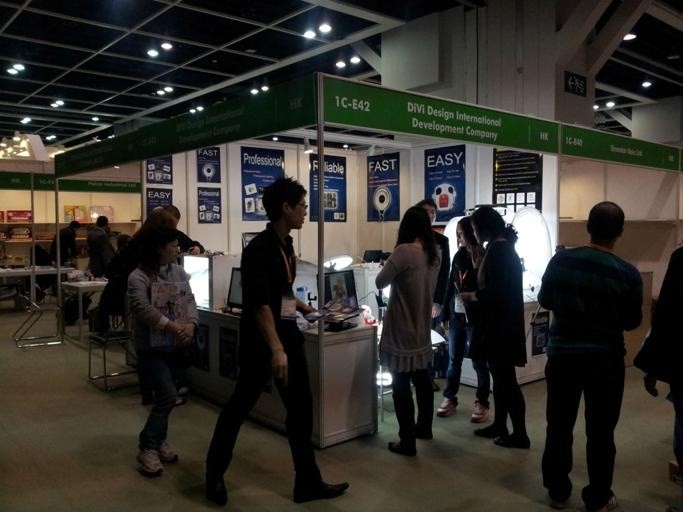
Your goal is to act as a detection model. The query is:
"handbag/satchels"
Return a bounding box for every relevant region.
[170,330,208,369]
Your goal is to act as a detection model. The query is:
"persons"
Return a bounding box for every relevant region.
[539,202,644,512]
[204,179,350,506]
[457,206,532,449]
[162,204,205,255]
[632,246,683,478]
[437,216,490,423]
[49,221,81,297]
[378,207,442,457]
[87,216,114,277]
[99,209,188,406]
[418,199,449,392]
[126,230,200,476]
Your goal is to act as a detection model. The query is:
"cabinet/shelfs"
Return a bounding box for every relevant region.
[0,222,137,285]
[624,272,653,368]
[124,307,378,450]
[445,298,553,392]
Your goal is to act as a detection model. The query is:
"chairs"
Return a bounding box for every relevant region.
[87,301,139,393]
[12,290,64,348]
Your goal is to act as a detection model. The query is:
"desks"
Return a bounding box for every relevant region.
[0,266,75,312]
[62,277,110,350]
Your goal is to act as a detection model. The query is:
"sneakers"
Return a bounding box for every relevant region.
[413,429,432,438]
[473,425,507,437]
[596,495,616,512]
[471,404,489,422]
[388,442,416,455]
[550,498,565,508]
[159,441,178,464]
[135,449,164,477]
[431,382,440,392]
[494,436,530,448]
[436,397,456,417]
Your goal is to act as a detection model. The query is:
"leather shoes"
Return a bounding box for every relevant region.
[293,481,349,503]
[204,471,228,505]
[174,399,186,406]
[178,387,195,396]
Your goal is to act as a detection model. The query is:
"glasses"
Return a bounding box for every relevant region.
[297,203,309,209]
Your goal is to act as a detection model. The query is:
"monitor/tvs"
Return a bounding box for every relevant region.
[222,267,243,316]
[316,268,359,333]
